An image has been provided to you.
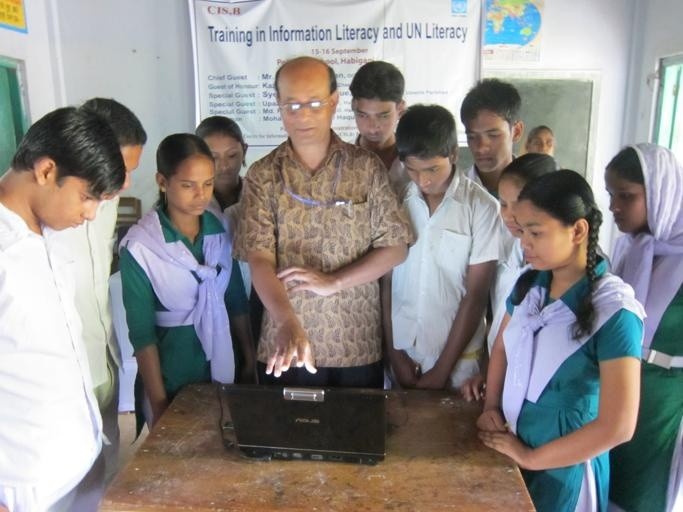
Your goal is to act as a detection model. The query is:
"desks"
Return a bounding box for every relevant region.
[100,384,538,511]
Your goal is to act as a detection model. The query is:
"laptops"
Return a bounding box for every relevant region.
[225,385,386,465]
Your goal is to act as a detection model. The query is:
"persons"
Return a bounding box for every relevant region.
[229,55,418,391]
[474,169,647,512]
[348,60,407,173]
[460,153,559,404]
[117,131,261,448]
[41,96,149,415]
[604,141,683,512]
[524,124,557,158]
[193,115,265,387]
[0,103,128,512]
[459,76,526,202]
[379,106,505,393]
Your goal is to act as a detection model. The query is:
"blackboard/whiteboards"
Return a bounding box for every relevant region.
[455,70,602,187]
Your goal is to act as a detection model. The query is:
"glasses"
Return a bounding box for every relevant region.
[278,98,334,113]
